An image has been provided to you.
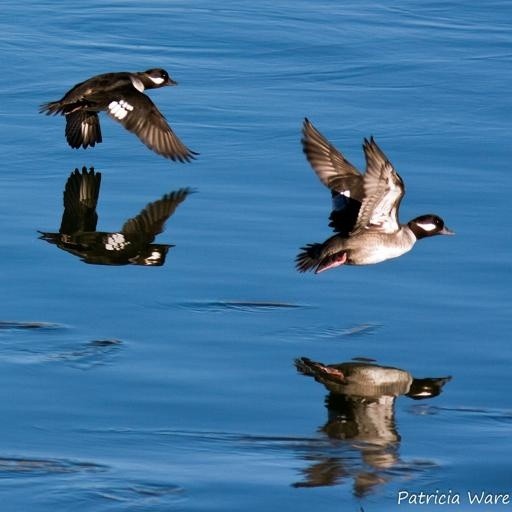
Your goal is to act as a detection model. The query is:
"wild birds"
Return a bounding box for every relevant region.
[292,116,457,276]
[287,355,452,499]
[36,165,199,267]
[37,67,202,165]
